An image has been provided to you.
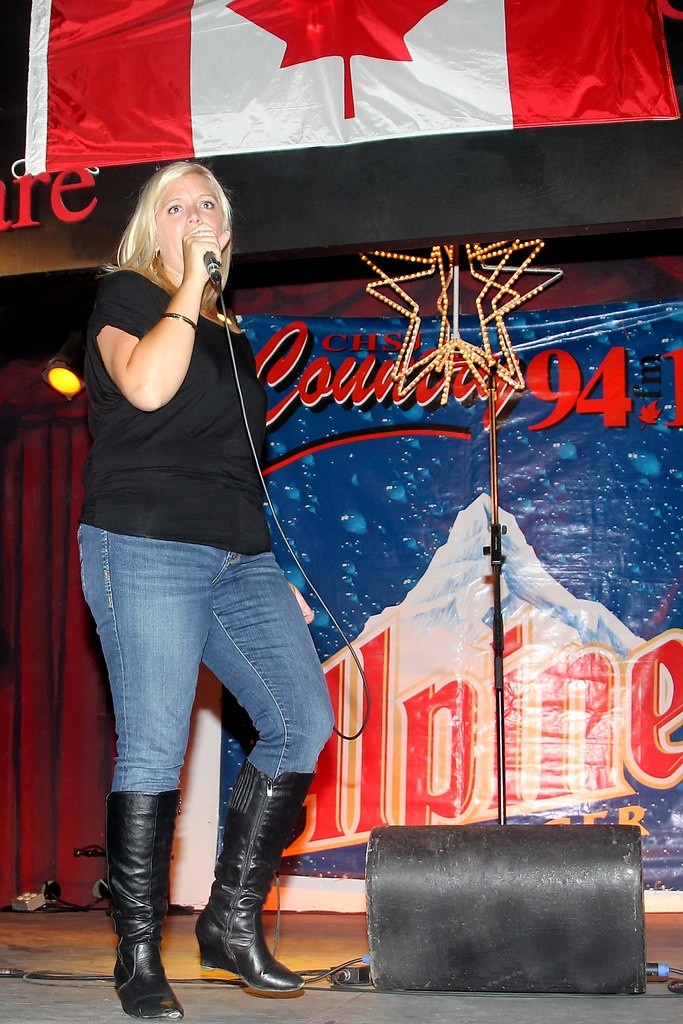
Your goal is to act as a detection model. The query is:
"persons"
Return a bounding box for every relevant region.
[77,162,336,1021]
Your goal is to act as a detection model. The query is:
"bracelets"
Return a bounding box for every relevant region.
[162,313,198,331]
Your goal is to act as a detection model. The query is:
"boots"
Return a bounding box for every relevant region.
[105,789,185,1020]
[195,757,315,993]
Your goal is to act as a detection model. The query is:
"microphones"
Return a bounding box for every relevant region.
[204,251,222,288]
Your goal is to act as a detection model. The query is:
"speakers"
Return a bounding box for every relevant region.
[365,822,649,994]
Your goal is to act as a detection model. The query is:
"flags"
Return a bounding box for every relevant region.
[25,0,681,176]
